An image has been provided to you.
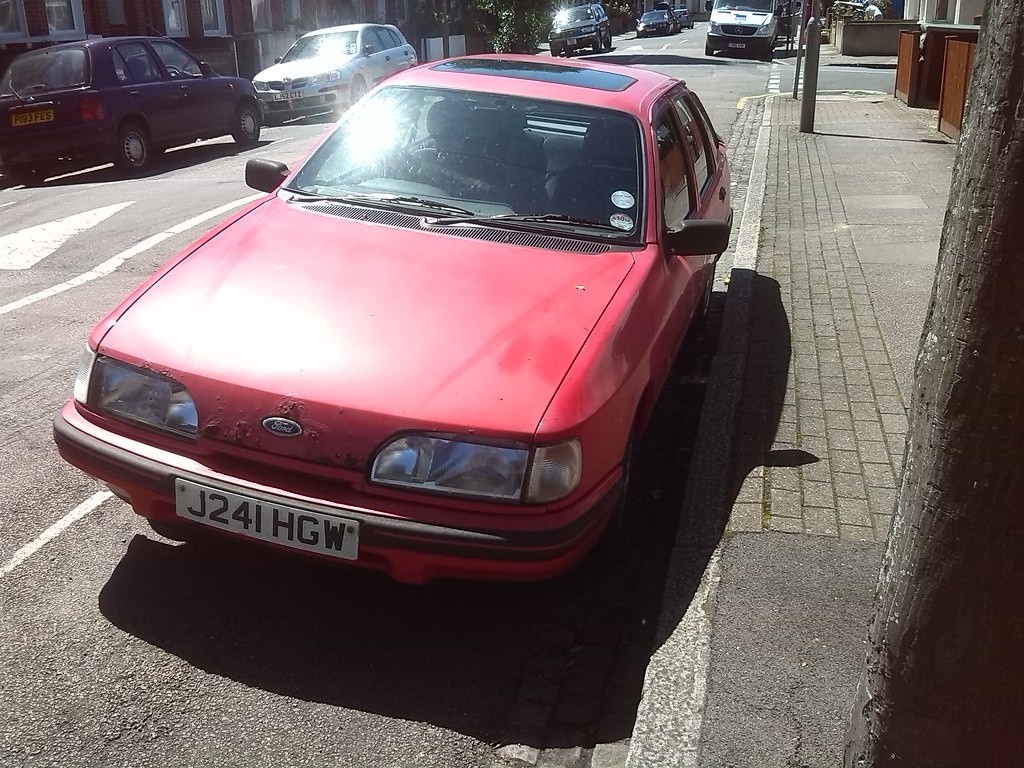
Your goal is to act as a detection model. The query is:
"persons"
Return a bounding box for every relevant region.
[866,5,883,21]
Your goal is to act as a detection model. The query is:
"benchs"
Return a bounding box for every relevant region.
[473,106,586,212]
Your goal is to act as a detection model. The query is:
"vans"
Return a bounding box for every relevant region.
[705,0,803,62]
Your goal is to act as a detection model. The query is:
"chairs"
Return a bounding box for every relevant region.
[124,58,149,83]
[568,119,638,227]
[412,97,496,199]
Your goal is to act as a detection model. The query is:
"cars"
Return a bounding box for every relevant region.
[674,8,695,30]
[636,10,682,37]
[54,52,735,584]
[548,4,612,57]
[0,36,267,187]
[251,23,418,128]
